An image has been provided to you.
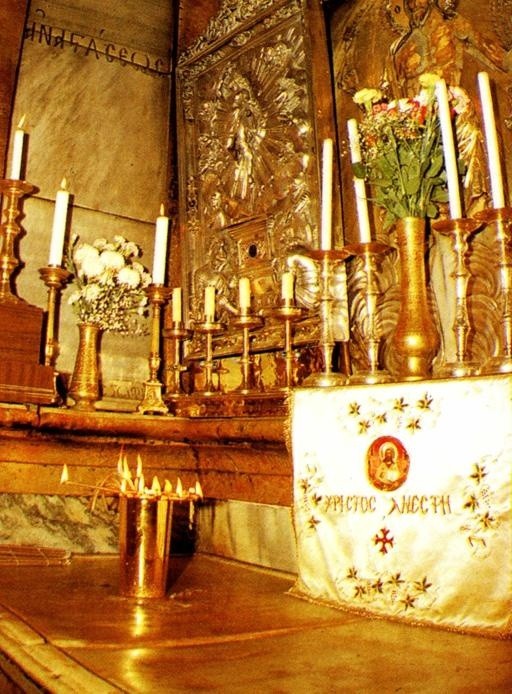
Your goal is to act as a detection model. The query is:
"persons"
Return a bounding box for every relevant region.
[225,89,253,200]
[374,448,406,484]
[337,0,511,112]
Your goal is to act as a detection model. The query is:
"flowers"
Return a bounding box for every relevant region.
[61,233,152,335]
[351,70,472,231]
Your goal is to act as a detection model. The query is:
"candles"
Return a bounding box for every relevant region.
[172,287,181,322]
[436,79,462,220]
[238,278,251,308]
[321,138,333,251]
[205,287,215,315]
[347,119,371,244]
[477,71,506,209]
[49,177,70,264]
[11,113,26,180]
[153,203,169,285]
[281,272,294,299]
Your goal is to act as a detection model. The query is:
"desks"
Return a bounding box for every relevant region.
[0,554,512,694]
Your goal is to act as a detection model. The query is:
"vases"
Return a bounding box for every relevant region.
[67,323,103,413]
[393,216,442,381]
